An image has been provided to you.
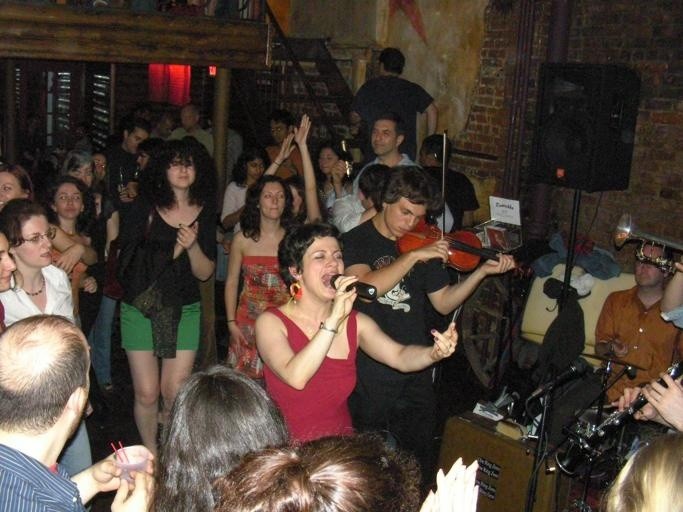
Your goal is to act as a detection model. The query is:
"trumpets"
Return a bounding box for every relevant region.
[613,208,683,277]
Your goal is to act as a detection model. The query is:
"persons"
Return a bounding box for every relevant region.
[0,46,683,512]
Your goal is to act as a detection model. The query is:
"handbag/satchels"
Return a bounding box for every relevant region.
[102,240,125,302]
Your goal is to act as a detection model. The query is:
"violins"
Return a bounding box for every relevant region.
[395,216,535,272]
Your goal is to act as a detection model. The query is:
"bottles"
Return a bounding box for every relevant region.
[340,139,354,180]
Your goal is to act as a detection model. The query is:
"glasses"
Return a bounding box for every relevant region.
[22,228,56,245]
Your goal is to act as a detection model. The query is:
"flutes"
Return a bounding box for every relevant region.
[556,361,683,475]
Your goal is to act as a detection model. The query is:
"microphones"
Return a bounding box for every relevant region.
[329,273,378,300]
[624,363,637,380]
[531,357,590,399]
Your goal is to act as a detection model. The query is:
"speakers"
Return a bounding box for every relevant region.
[525,61,642,194]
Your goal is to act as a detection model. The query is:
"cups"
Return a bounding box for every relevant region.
[117,163,141,194]
[110,443,151,488]
